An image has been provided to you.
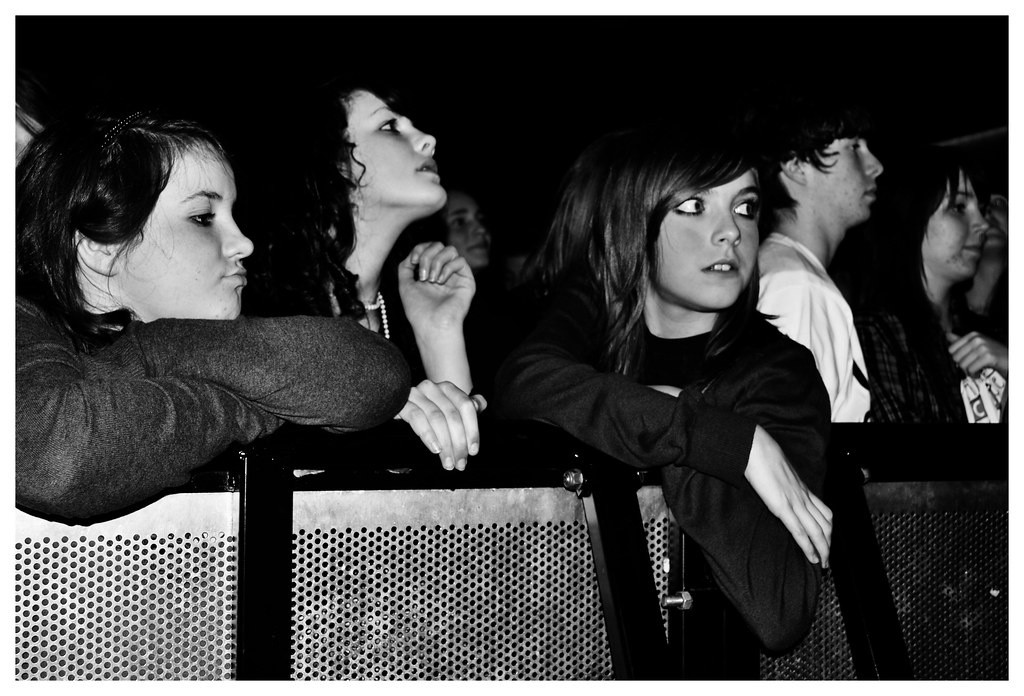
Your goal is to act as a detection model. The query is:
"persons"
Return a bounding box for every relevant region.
[437,167,547,322]
[244,76,491,475]
[15,110,416,525]
[494,123,834,654]
[730,67,886,426]
[942,168,1009,348]
[839,140,1009,425]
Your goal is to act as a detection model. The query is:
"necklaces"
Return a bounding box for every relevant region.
[323,275,390,342]
